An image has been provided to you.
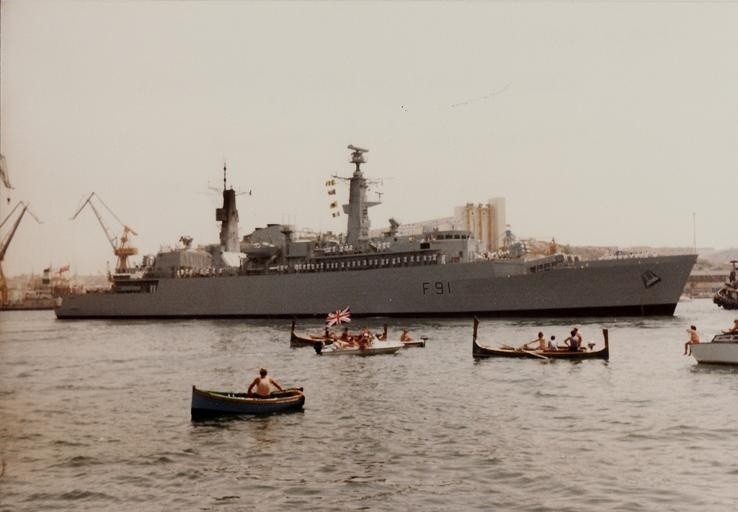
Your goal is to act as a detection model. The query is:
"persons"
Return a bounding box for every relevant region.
[309,325,386,352]
[523,328,582,352]
[721,319,738,334]
[247,368,283,399]
[400,328,413,341]
[683,325,699,356]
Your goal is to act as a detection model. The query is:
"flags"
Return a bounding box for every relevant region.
[324,305,352,329]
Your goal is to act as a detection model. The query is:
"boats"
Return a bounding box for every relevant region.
[190,384,305,421]
[289,323,425,356]
[712,261,738,312]
[471,316,610,361]
[689,335,737,365]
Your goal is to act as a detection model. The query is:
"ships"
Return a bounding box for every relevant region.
[0,266,86,310]
[54,144,698,316]
